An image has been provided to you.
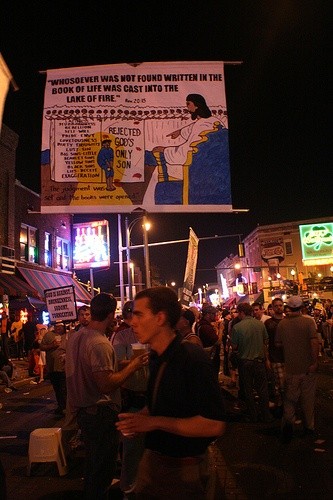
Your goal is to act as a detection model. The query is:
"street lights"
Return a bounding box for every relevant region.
[124,210,153,300]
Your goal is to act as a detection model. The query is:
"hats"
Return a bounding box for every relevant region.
[287,295,301,308]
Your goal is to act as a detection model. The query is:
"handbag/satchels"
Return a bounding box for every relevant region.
[203,345,216,359]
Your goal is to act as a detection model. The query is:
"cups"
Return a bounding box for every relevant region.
[131,344,147,358]
[118,413,135,435]
[54,335,61,344]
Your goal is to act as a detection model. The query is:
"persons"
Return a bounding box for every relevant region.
[0,298,150,498]
[115,287,225,500]
[65,292,151,500]
[175,295,333,442]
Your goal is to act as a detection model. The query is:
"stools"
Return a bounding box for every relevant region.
[26,428,68,475]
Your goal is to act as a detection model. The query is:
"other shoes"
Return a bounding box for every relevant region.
[38,378,45,384]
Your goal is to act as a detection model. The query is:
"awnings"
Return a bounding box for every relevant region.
[15,266,93,303]
[0,273,38,296]
